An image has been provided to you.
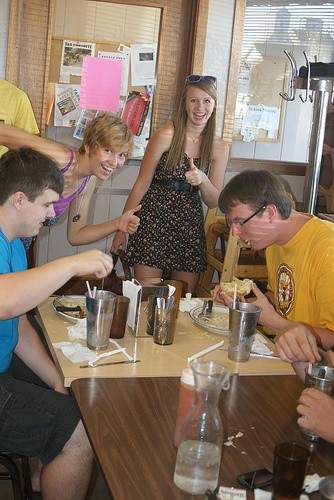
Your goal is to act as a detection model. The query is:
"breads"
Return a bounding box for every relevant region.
[221,277,253,298]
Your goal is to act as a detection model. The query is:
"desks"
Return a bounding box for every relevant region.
[69,375,334,500]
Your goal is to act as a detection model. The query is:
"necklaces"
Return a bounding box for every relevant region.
[72,156,88,222]
[186,127,200,143]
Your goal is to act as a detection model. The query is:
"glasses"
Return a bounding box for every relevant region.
[183,74,218,90]
[226,203,267,227]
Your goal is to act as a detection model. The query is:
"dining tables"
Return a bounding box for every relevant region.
[35,296,295,387]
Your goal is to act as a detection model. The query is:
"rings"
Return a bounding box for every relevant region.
[192,180,194,183]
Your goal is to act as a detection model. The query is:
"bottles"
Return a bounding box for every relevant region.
[172,360,229,496]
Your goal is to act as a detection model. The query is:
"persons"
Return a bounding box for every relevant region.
[0,113,142,254]
[110,75,229,299]
[0,149,113,500]
[211,169,334,352]
[273,320,334,445]
[0,77,40,159]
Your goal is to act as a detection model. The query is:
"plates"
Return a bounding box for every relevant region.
[51,295,87,321]
[189,305,229,336]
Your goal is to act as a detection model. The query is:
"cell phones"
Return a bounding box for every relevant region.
[238,467,275,488]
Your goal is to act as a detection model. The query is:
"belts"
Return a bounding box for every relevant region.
[152,178,199,193]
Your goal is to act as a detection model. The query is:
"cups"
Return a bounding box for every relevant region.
[153,307,177,346]
[164,280,190,320]
[146,295,166,336]
[302,366,334,443]
[271,442,310,500]
[229,301,262,362]
[318,475,334,500]
[84,290,117,350]
[108,296,130,339]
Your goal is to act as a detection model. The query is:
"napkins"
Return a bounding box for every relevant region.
[179,291,204,312]
[50,340,97,364]
[67,317,87,339]
[250,332,274,356]
